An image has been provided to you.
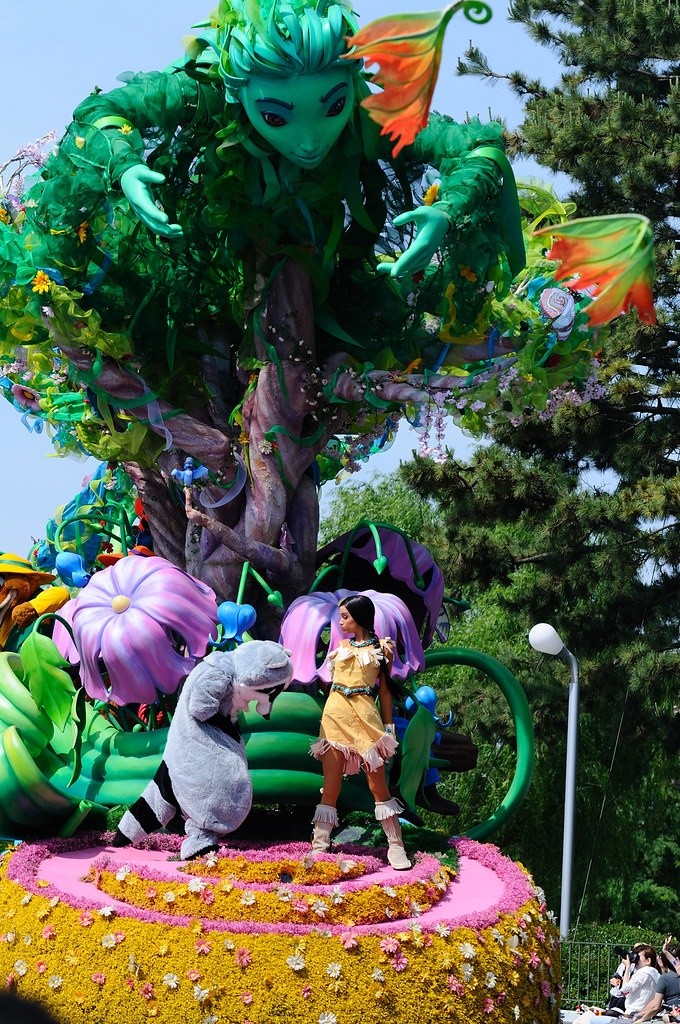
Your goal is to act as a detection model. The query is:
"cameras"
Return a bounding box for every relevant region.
[614,945,640,962]
[661,1004,678,1012]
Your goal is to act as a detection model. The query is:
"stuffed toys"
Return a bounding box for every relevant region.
[0,553,70,651]
[112,640,294,861]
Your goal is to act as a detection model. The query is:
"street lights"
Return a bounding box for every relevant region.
[527,619,583,941]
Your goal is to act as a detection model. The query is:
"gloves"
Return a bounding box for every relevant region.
[384,723,397,741]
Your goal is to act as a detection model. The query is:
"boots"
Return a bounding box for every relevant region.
[312,804,339,854]
[374,796,412,870]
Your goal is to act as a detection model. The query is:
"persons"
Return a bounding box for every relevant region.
[309,594,412,870]
[602,935,680,1024]
[72,0,510,279]
